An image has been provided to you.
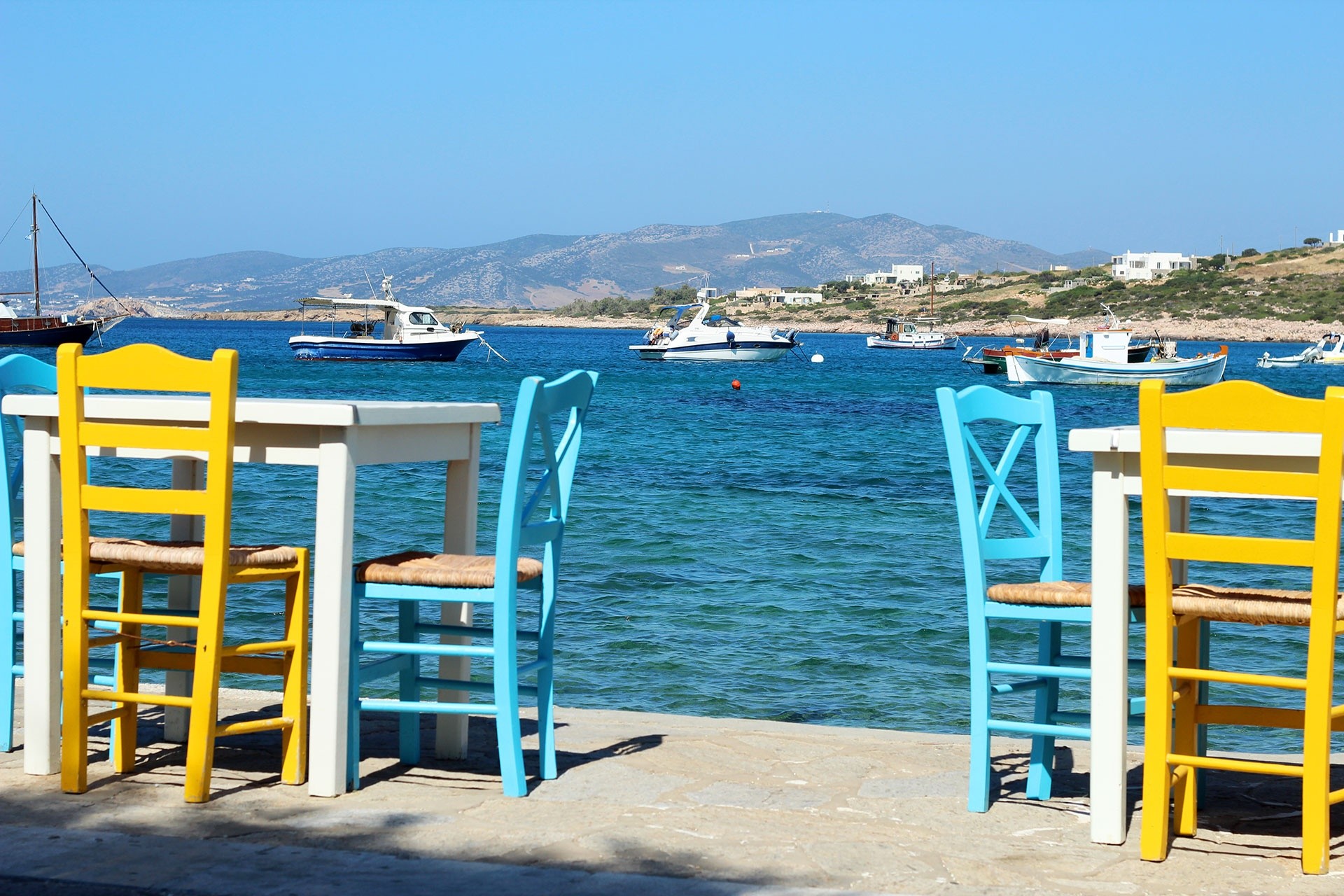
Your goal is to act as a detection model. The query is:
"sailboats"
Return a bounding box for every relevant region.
[0,185,131,347]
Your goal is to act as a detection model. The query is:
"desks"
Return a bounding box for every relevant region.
[1069,426,1322,845]
[1,395,501,796]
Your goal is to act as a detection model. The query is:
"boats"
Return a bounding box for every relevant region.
[1303,331,1344,366]
[289,268,484,362]
[1257,351,1306,368]
[866,315,959,349]
[1001,323,1230,388]
[627,274,802,363]
[961,314,1153,376]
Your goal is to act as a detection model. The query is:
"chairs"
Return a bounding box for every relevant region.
[937,385,1211,813]
[1139,380,1343,874]
[0,354,181,764]
[56,342,310,804]
[345,370,600,799]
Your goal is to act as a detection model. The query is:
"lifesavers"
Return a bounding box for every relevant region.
[892,334,898,340]
